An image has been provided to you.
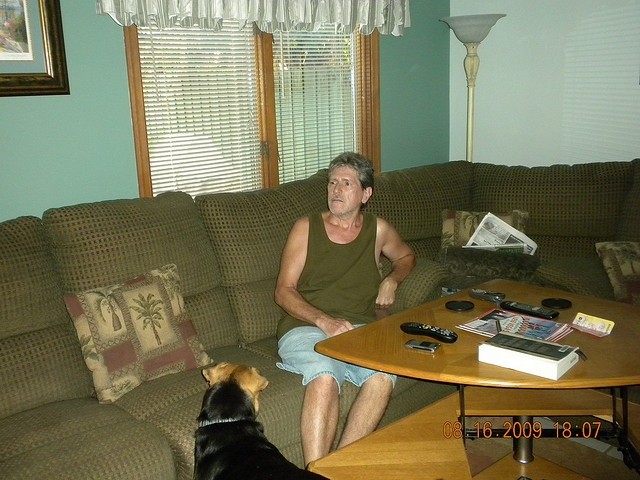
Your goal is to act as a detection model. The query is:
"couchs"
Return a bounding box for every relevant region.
[0,159,640,479]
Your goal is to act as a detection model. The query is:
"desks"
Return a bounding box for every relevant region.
[308,278,639,478]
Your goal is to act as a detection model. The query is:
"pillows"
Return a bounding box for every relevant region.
[597,240,640,302]
[62,263,214,407]
[444,248,540,295]
[437,207,531,259]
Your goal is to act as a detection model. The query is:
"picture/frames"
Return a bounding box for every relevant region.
[0,0,71,96]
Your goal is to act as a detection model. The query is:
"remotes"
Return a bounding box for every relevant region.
[469,289,505,303]
[400,321,458,343]
[500,301,559,321]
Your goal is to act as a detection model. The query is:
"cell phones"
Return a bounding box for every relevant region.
[405,339,439,353]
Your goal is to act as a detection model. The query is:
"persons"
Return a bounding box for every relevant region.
[274,152,416,469]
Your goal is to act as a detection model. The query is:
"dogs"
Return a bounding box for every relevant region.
[191,359,331,480]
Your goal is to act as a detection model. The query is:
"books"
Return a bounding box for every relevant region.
[477,332,584,382]
[454,307,574,344]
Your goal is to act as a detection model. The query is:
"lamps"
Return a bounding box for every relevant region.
[94,1,411,38]
[440,14,505,161]
